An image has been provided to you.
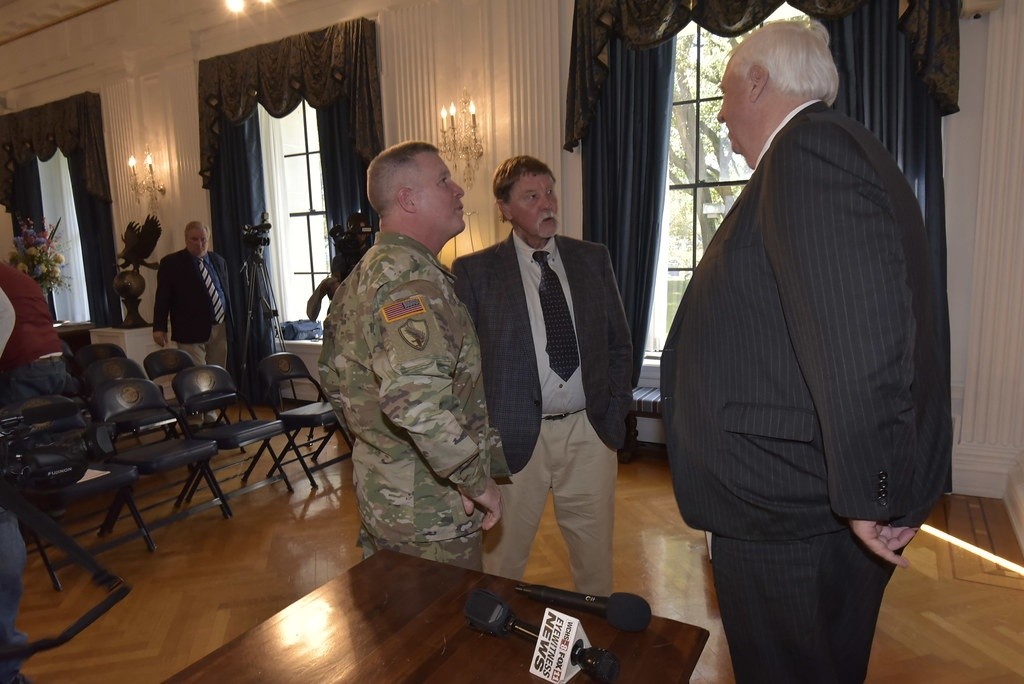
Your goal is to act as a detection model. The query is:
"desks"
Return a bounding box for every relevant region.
[163,548,709,684]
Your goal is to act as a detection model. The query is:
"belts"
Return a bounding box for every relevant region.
[541,408,586,420]
[35,356,64,362]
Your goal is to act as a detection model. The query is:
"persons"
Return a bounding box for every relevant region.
[152,221,234,430]
[329,210,375,300]
[0,260,67,447]
[660,19,953,684]
[0,286,29,684]
[319,140,511,573]
[449,156,632,599]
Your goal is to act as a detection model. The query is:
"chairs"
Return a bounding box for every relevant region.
[2,340,357,592]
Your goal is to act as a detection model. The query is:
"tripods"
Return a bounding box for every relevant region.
[239,243,299,422]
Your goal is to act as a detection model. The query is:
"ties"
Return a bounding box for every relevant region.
[533,251,579,382]
[198,258,224,324]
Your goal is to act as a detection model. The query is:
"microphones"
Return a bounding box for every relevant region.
[254,224,272,231]
[514,585,651,632]
[463,590,620,684]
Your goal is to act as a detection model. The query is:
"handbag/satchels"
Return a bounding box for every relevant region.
[276,319,322,340]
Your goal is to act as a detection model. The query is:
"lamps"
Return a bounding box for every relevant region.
[439,96,483,185]
[125,145,165,202]
